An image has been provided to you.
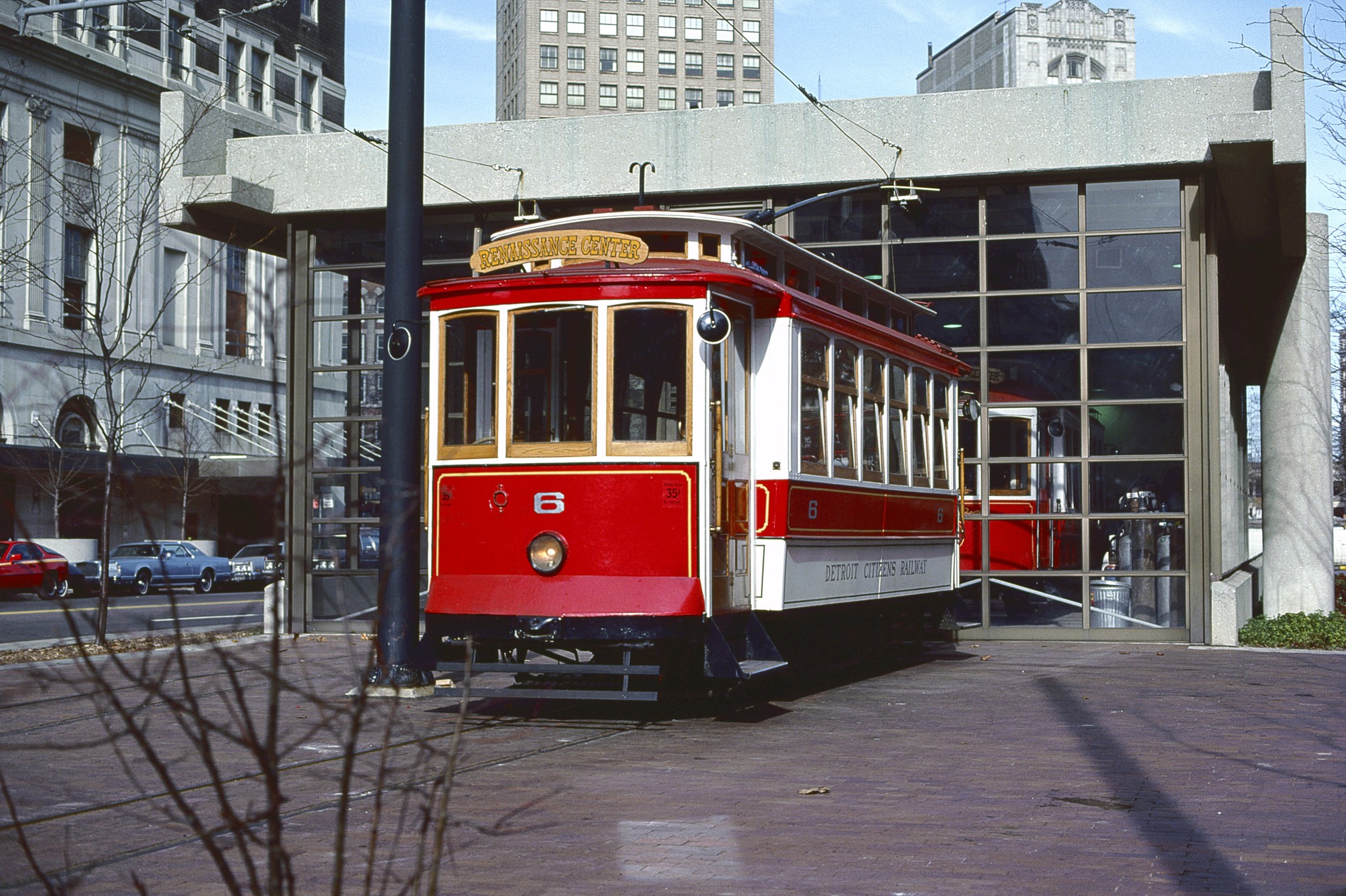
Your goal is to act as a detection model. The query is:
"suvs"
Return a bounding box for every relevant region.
[312,528,381,569]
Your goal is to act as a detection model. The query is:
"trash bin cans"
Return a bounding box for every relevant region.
[1087,578,1133,629]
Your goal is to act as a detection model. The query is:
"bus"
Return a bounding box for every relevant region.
[414,177,973,702]
[950,391,1109,581]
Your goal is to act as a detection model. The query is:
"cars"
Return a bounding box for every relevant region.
[228,543,285,586]
[0,540,70,600]
[73,540,233,596]
